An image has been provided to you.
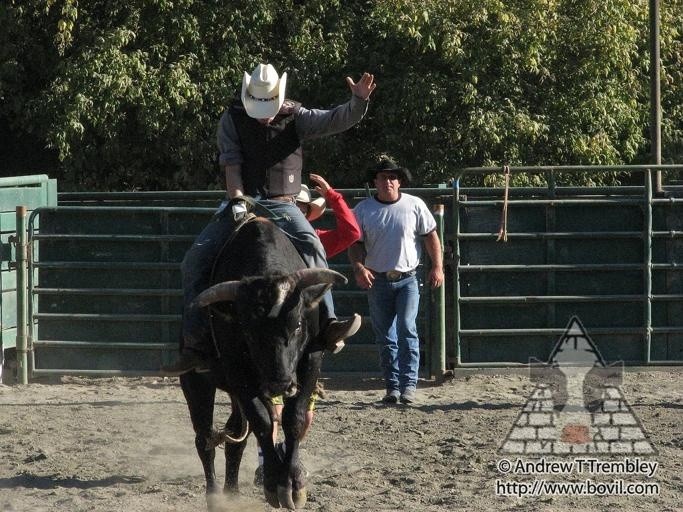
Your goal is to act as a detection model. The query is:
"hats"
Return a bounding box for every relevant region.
[296,184,327,222]
[241,64,288,119]
[366,160,411,184]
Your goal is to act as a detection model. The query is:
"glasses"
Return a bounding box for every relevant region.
[380,175,396,180]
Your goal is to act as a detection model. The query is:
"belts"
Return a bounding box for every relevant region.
[376,272,410,281]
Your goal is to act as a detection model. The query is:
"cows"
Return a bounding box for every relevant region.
[179,213,326,510]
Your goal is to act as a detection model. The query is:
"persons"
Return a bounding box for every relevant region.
[158,56,379,376]
[251,172,361,489]
[345,157,445,405]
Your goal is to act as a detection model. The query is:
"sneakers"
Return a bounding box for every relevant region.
[400,392,414,403]
[253,466,263,486]
[383,393,397,403]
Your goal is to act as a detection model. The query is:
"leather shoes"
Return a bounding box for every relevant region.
[160,348,210,376]
[324,313,361,354]
